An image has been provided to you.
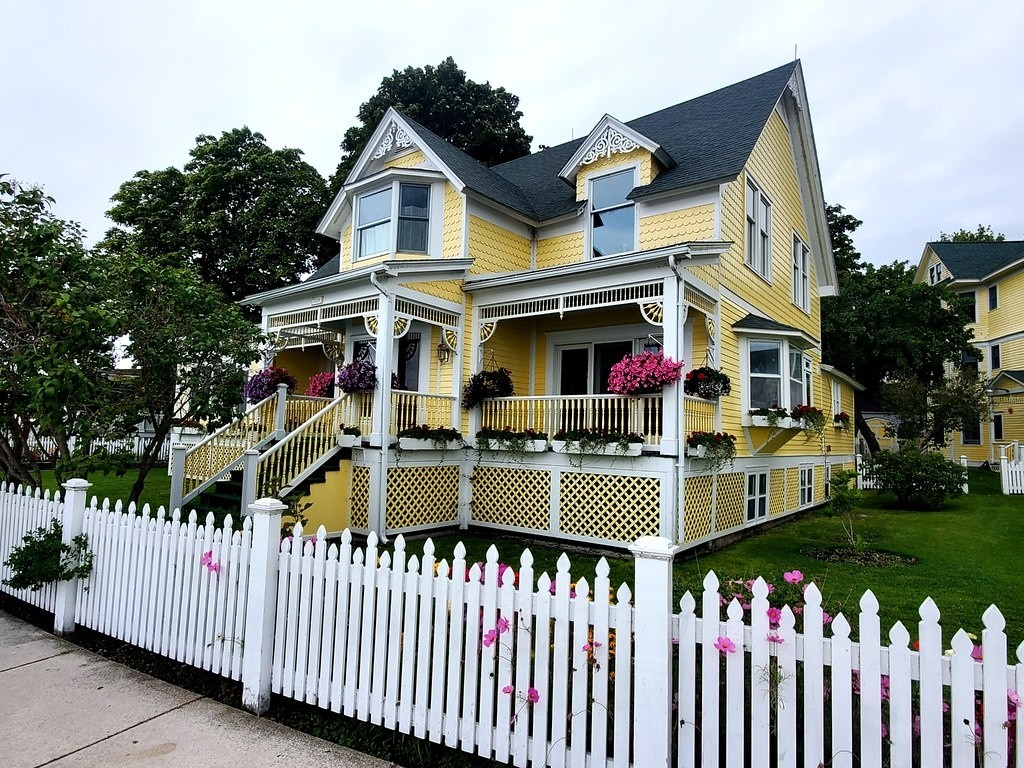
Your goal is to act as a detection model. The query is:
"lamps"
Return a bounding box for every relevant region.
[436,342,449,365]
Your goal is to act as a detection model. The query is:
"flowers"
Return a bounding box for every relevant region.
[462,367,518,415]
[395,421,468,469]
[304,373,335,396]
[684,366,732,400]
[336,357,379,393]
[607,348,685,397]
[392,372,408,390]
[243,364,298,405]
[687,431,738,475]
[340,423,362,438]
[834,412,851,437]
[554,426,645,471]
[472,425,548,467]
[174,421,205,440]
[749,404,826,454]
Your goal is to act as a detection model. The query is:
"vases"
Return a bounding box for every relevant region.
[550,440,643,457]
[474,438,548,452]
[172,427,205,435]
[398,437,464,450]
[687,444,725,457]
[834,423,844,429]
[338,435,362,448]
[751,415,810,430]
